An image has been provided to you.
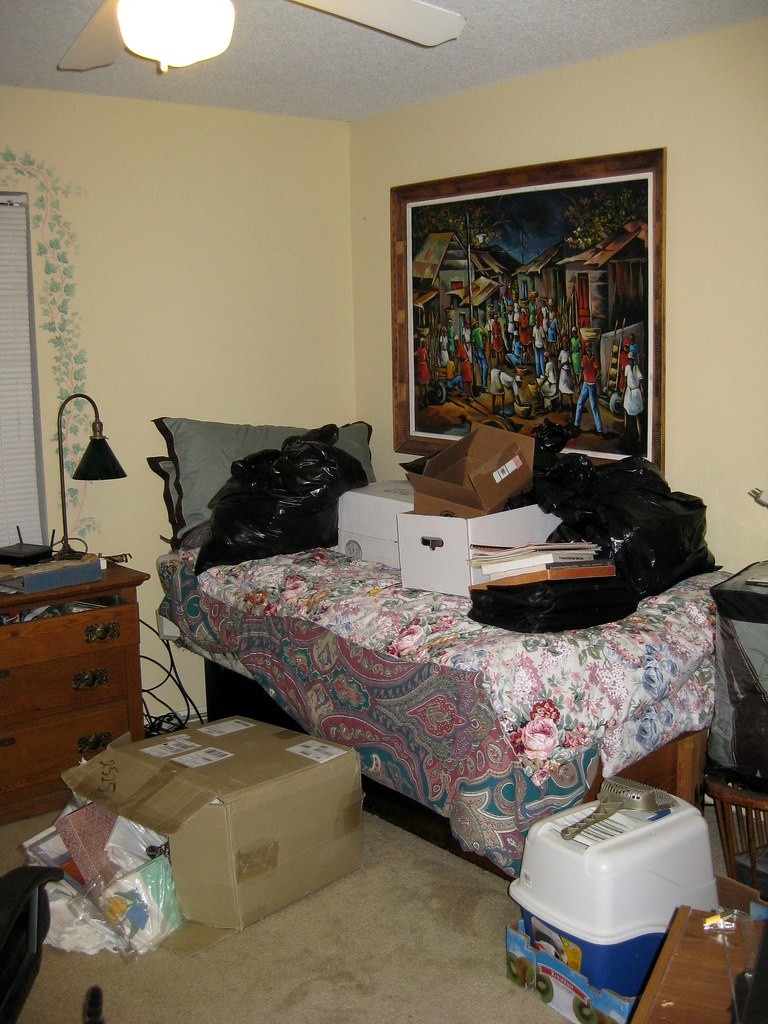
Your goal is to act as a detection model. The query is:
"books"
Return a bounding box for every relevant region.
[466,542,616,596]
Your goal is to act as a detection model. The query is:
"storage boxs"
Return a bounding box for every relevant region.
[395,504,563,597]
[59,715,366,960]
[437,421,536,489]
[337,480,414,570]
[505,920,768,1024]
[398,455,534,519]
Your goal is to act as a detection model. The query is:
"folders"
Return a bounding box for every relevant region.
[0,562,104,595]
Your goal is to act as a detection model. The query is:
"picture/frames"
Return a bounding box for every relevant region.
[389,148,666,478]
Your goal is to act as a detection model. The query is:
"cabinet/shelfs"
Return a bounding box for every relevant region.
[0,556,152,825]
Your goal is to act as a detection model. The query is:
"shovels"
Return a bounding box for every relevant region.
[560,775,678,840]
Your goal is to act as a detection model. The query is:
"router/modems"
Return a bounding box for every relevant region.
[0,526,56,566]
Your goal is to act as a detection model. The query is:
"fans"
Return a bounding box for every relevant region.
[57,0,466,72]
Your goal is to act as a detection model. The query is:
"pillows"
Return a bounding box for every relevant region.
[146,456,180,552]
[151,416,376,550]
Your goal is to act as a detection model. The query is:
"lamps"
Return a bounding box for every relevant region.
[117,0,236,72]
[52,394,127,560]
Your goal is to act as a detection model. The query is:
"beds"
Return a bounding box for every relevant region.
[149,416,734,880]
